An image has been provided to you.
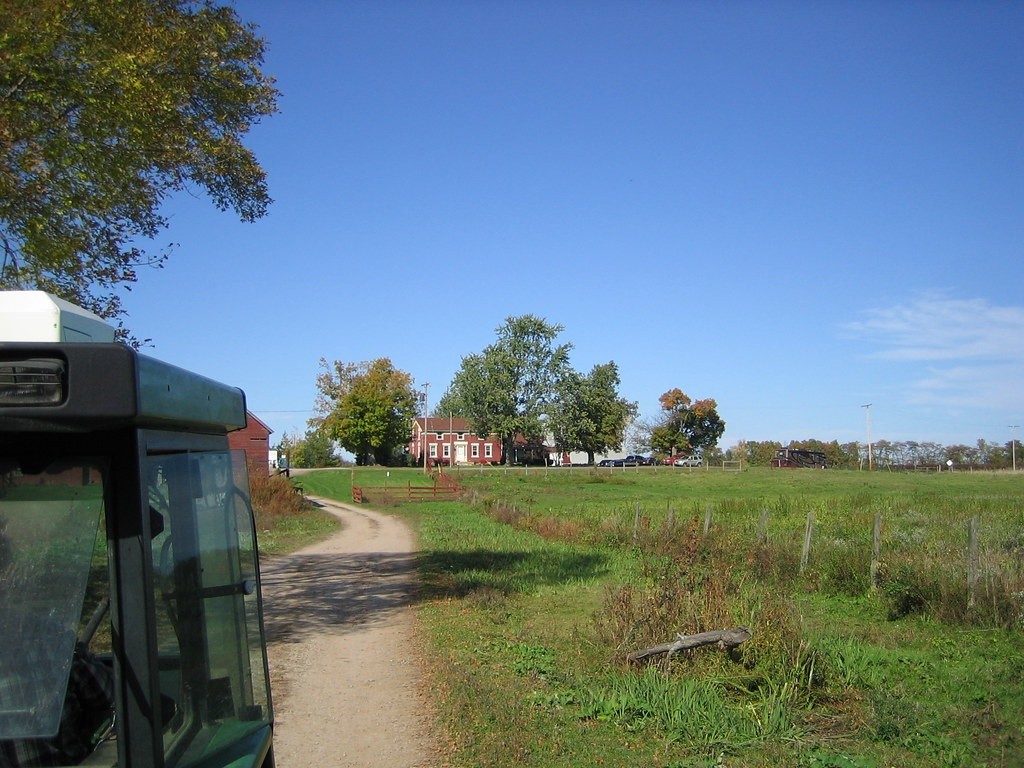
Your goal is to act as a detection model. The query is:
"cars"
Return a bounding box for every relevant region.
[674,454,705,468]
[598,454,659,467]
[664,453,687,466]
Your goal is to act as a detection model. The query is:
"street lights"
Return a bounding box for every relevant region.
[421,381,431,475]
